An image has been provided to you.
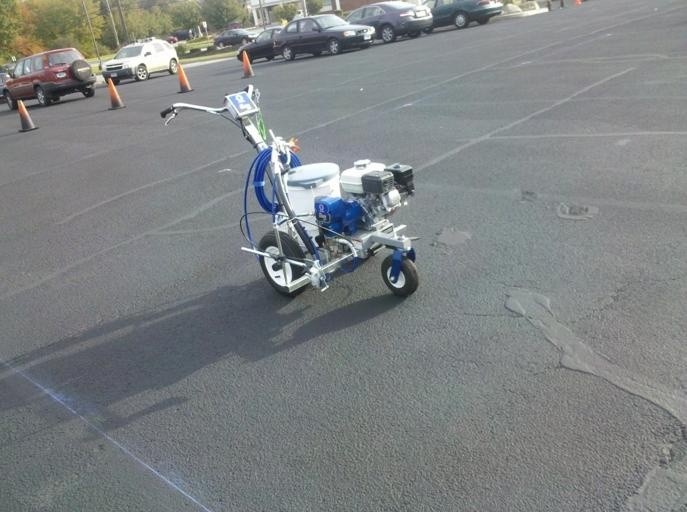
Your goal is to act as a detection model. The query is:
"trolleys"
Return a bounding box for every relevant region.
[159,86,420,298]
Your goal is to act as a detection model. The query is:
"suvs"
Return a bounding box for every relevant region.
[5,47,97,107]
[100,40,179,86]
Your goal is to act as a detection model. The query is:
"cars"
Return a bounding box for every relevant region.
[346,0,433,44]
[166,37,178,44]
[273,13,377,61]
[213,29,257,48]
[237,26,287,64]
[425,0,504,32]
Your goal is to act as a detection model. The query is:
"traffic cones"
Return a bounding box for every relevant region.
[15,100,39,132]
[175,63,196,93]
[105,78,126,110]
[237,50,257,79]
